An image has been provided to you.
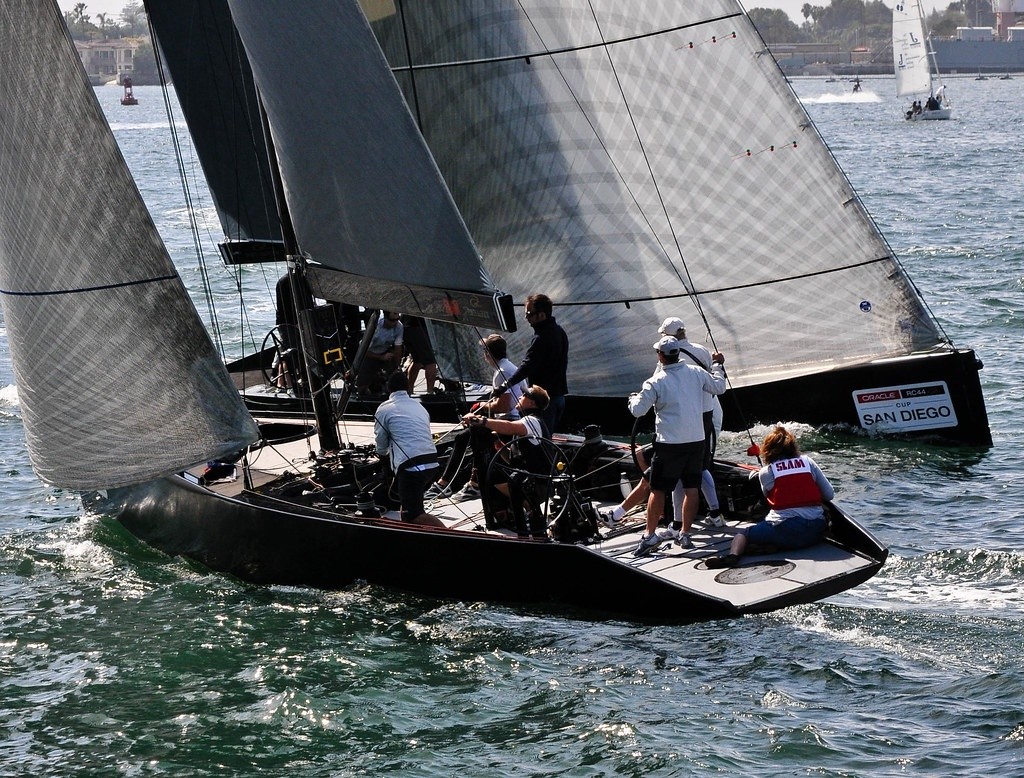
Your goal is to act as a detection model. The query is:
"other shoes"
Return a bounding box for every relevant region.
[705,554,741,568]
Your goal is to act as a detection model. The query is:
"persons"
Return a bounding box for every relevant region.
[356,311,436,395]
[925,97,939,110]
[463,384,555,539]
[422,334,527,502]
[705,427,834,570]
[936,84,946,104]
[907,101,921,116]
[593,315,725,540]
[852,81,862,93]
[628,336,726,559]
[373,372,446,528]
[491,294,569,435]
[275,272,314,395]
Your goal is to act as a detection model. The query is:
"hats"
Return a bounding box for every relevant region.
[658,317,686,336]
[521,384,550,409]
[479,334,506,353]
[653,336,680,355]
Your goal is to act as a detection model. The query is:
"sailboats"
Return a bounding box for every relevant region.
[1,0,996,625]
[892,0,953,121]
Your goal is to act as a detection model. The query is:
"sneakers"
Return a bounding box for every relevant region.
[452,482,482,501]
[705,512,726,527]
[595,508,624,530]
[658,522,681,539]
[674,528,693,550]
[423,482,452,499]
[633,532,661,556]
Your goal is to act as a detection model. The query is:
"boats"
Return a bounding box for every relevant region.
[973,76,989,80]
[826,78,839,83]
[121,77,140,107]
[999,73,1014,80]
[848,77,863,82]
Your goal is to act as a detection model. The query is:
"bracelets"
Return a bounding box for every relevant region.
[481,416,487,426]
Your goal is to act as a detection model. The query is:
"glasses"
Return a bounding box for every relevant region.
[525,310,542,318]
[388,318,400,322]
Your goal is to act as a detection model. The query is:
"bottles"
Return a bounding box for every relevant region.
[619,472,633,499]
